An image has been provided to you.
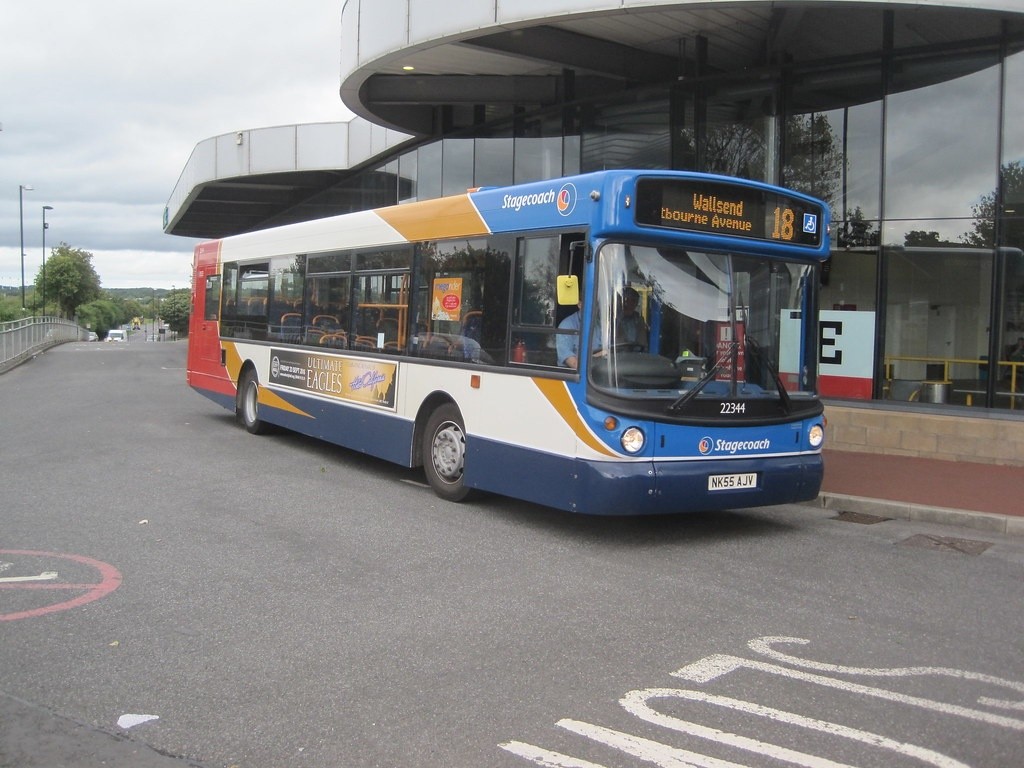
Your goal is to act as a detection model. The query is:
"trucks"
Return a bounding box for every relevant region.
[106,329,128,343]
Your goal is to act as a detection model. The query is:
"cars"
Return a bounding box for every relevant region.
[407,331,480,363]
[89,332,99,342]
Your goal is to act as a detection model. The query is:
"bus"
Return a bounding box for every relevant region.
[187,169,831,517]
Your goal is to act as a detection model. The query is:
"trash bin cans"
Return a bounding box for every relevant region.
[922,381,953,403]
[159,329,166,342]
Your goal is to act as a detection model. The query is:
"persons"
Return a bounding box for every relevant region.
[555,287,651,371]
[1009,338,1024,373]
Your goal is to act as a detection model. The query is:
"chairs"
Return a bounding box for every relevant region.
[226,297,486,364]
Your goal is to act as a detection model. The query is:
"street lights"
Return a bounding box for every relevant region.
[19,185,34,308]
[152,288,157,341]
[172,285,175,340]
[42,206,53,321]
